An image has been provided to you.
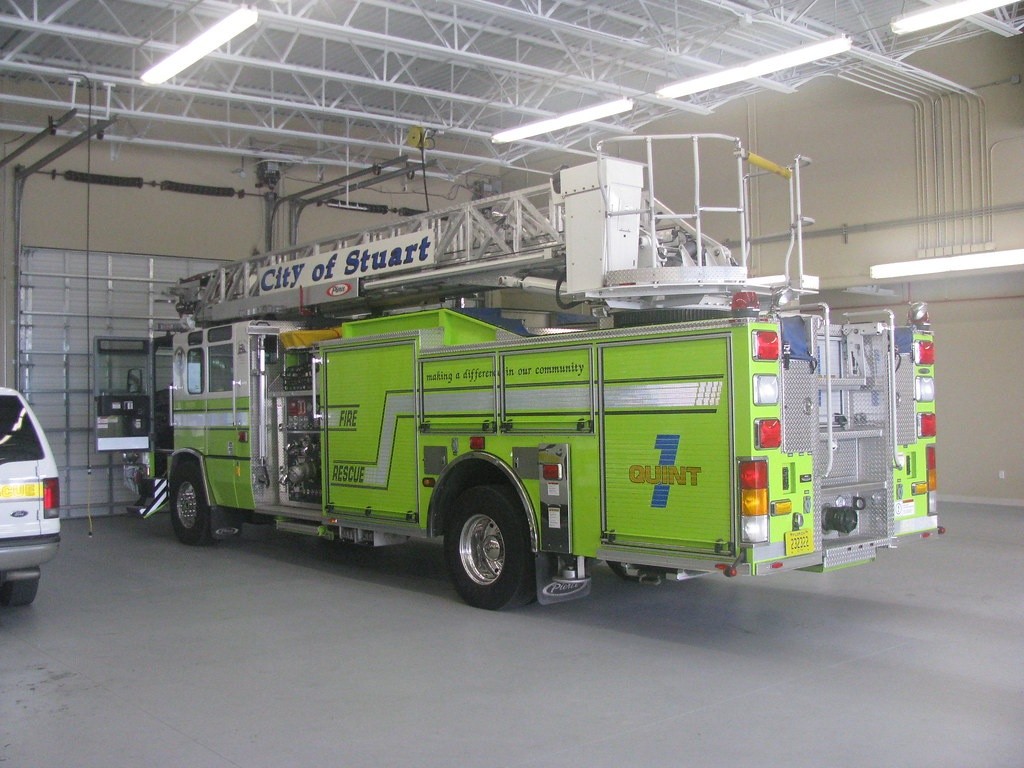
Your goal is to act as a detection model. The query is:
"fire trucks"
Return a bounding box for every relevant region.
[93,132,944,609]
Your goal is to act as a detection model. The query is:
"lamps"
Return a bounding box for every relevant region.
[654,33,852,99]
[890,0,1024,35]
[137,0,258,84]
[490,94,633,144]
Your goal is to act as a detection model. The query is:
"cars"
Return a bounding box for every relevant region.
[0,385,63,608]
